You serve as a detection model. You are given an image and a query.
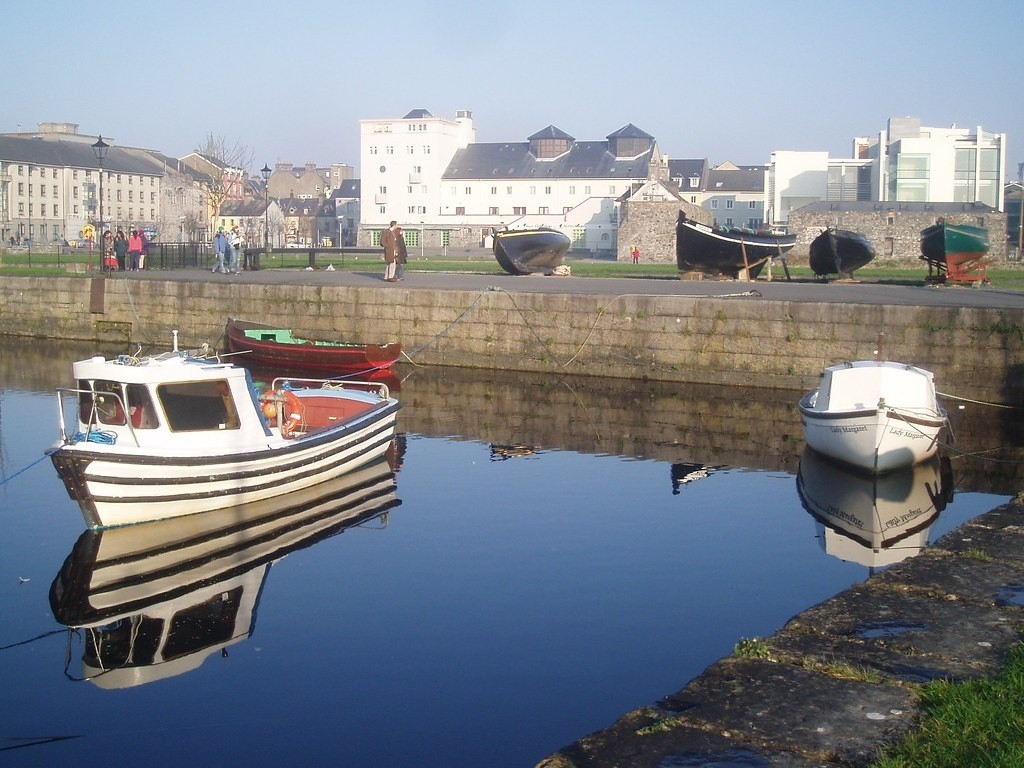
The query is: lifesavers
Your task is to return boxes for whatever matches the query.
[257,389,302,431]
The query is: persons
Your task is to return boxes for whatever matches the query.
[103,229,147,272]
[633,247,639,264]
[380,221,409,282]
[9,234,21,246]
[211,225,241,276]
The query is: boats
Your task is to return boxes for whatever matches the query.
[490,225,571,275]
[41,326,403,532]
[917,214,992,275]
[674,209,798,281]
[798,360,951,473]
[809,227,876,279]
[226,354,397,392]
[795,443,953,567]
[225,315,403,371]
[48,454,404,689]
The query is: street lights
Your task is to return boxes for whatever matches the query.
[90,134,109,273]
[419,220,425,255]
[260,163,272,247]
[337,215,344,255]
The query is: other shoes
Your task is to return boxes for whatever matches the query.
[384,278,404,282]
[236,271,240,273]
[226,268,229,272]
[212,268,215,272]
[221,270,226,274]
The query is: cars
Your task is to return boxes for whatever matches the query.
[75,239,97,248]
[283,240,311,248]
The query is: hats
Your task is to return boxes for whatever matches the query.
[218,226,224,230]
[139,230,144,234]
